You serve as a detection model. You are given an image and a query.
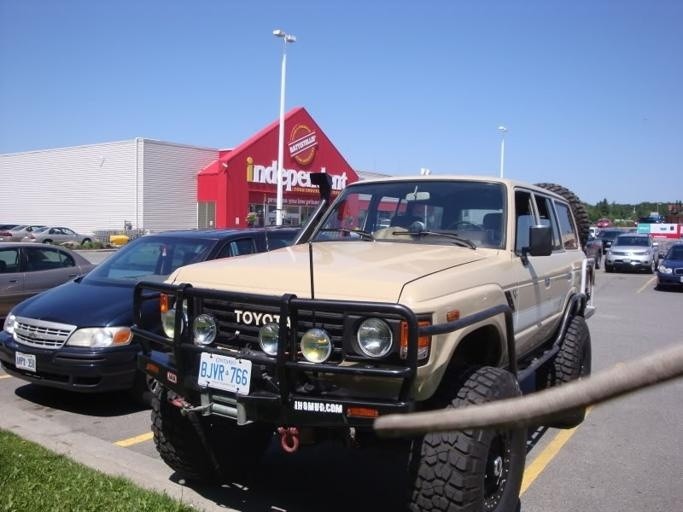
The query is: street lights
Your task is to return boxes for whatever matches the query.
[273,29,296,225]
[496,127,508,179]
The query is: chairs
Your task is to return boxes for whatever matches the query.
[483,213,501,243]
[391,216,423,229]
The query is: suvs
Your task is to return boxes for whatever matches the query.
[130,169,595,511]
[0,227,330,409]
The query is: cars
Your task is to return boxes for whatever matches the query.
[0,224,19,231]
[0,226,50,242]
[0,241,92,321]
[586,223,683,290]
[19,227,95,248]
